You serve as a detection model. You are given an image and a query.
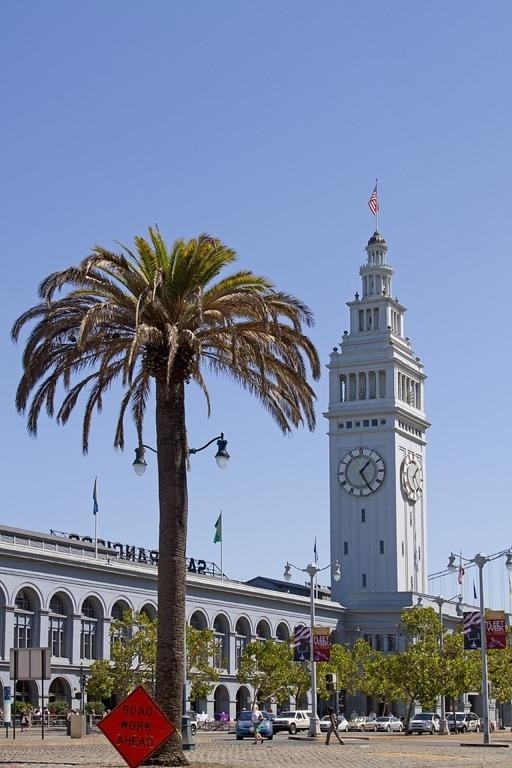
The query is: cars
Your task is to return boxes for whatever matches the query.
[347,716,378,732]
[320,714,349,733]
[479,719,494,733]
[398,716,412,722]
[234,711,275,740]
[268,713,277,723]
[445,712,457,719]
[374,717,403,733]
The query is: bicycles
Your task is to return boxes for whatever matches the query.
[210,713,231,731]
[196,717,212,730]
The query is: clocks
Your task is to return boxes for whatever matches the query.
[400,454,423,504]
[337,447,385,497]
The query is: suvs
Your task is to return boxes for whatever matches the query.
[187,711,197,736]
[272,710,321,735]
[445,712,480,734]
[408,713,441,734]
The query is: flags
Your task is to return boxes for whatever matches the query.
[474,585,477,598]
[214,514,222,544]
[368,184,378,215]
[314,540,318,560]
[93,480,99,515]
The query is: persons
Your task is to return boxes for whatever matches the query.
[325,707,345,745]
[251,704,264,745]
[19,706,50,732]
[350,709,394,719]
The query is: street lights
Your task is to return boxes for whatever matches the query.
[132,432,231,750]
[446,547,511,750]
[414,593,465,736]
[284,559,341,738]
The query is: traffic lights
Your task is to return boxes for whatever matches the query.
[81,674,86,687]
[324,673,336,692]
